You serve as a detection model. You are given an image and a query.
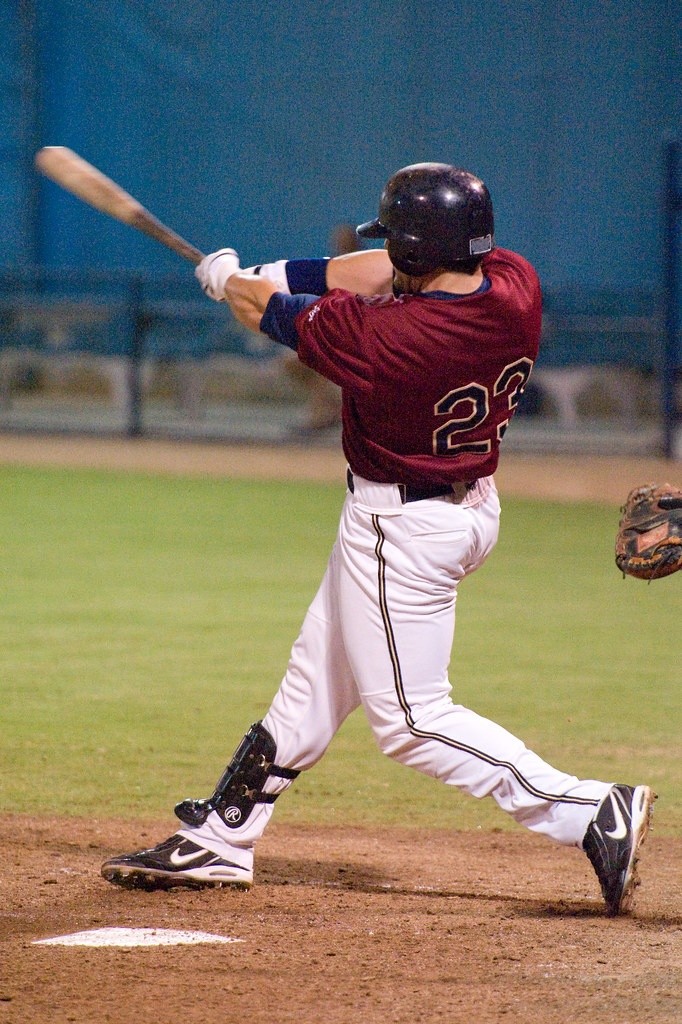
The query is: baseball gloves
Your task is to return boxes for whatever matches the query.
[613,483,681,582]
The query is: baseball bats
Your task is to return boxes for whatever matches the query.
[35,144,206,264]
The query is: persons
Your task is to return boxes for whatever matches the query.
[102,161,658,914]
[285,224,363,427]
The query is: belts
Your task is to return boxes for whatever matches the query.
[346,468,475,504]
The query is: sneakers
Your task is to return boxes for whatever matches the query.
[101,835,254,890]
[582,785,659,914]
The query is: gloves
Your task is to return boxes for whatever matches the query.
[194,248,291,302]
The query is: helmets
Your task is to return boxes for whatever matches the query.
[356,162,495,277]
